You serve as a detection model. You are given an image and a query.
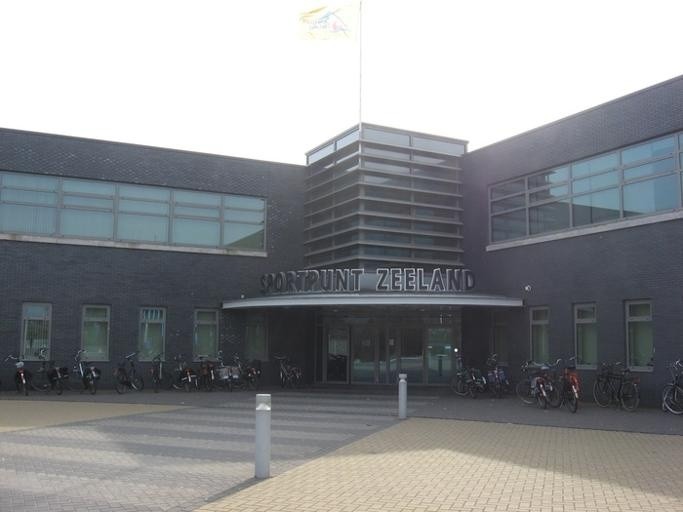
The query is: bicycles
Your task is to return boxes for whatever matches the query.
[5,352,28,395]
[74,349,99,396]
[446,346,683,417]
[172,350,262,394]
[30,347,69,395]
[113,350,146,395]
[276,357,302,390]
[150,351,167,393]
